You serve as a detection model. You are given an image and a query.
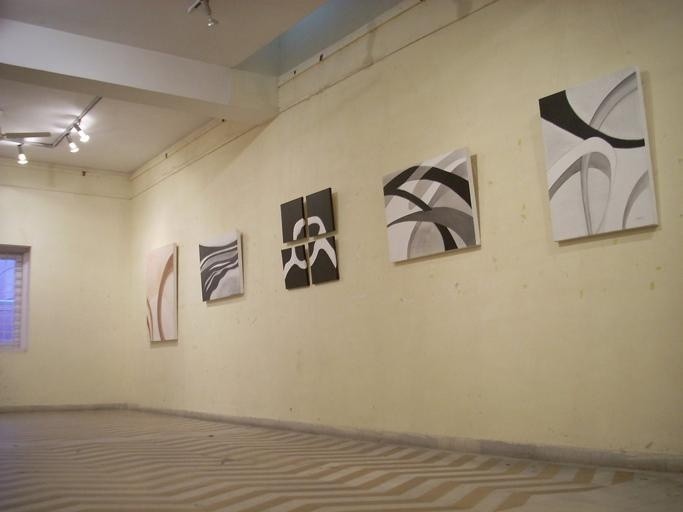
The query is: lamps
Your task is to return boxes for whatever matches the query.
[200,1,218,32]
